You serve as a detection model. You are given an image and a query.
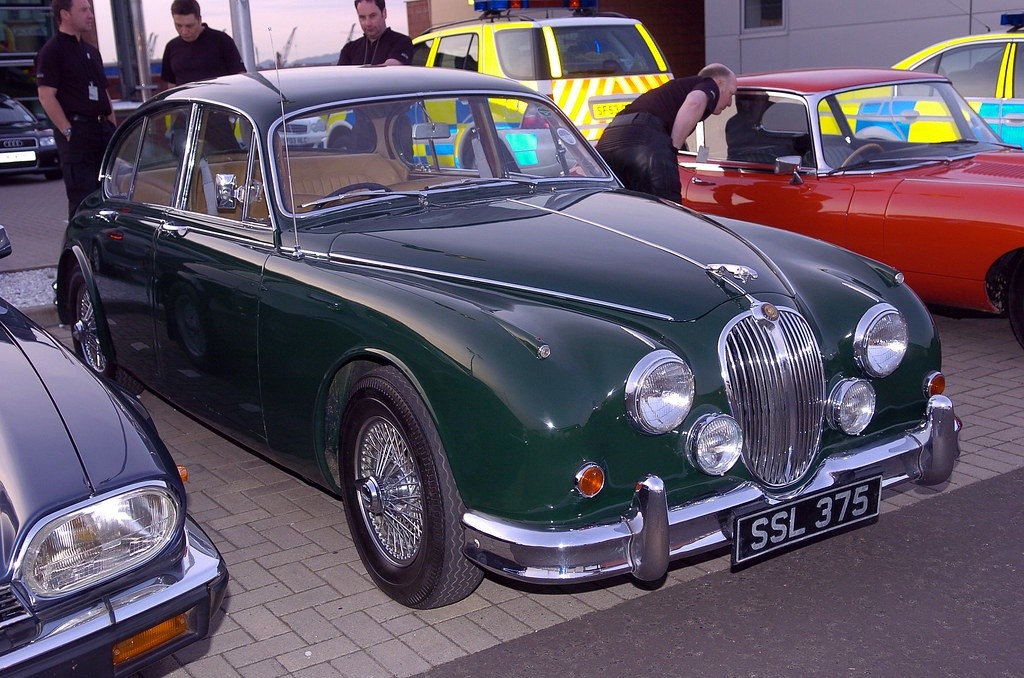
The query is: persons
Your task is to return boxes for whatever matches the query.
[596,62,737,207]
[725,85,806,164]
[160,0,247,159]
[33,0,117,221]
[338,0,415,165]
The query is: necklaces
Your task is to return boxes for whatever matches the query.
[362,37,381,65]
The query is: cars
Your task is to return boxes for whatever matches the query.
[278,114,330,150]
[55,63,960,611]
[0,91,65,182]
[676,69,1024,352]
[0,224,229,678]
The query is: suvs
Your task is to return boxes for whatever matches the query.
[323,0,679,178]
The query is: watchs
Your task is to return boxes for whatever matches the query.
[63,127,72,136]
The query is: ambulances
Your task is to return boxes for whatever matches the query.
[819,11,1024,148]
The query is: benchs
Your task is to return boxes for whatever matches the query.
[116,152,409,223]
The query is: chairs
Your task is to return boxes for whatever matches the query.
[823,146,865,168]
[748,145,781,165]
